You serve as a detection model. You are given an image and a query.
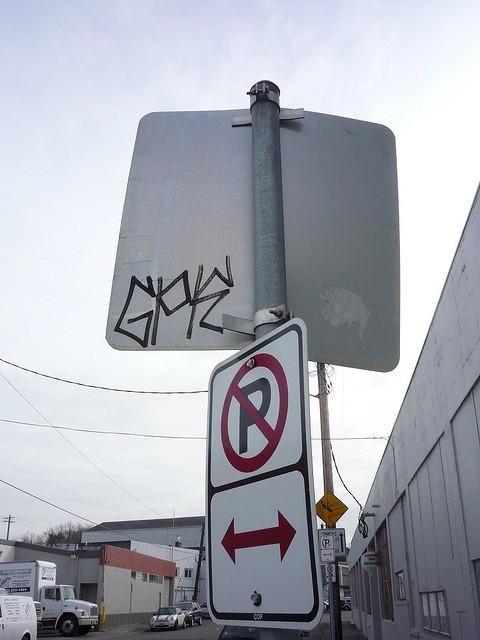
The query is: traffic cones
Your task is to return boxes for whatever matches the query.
[100,605,106,631]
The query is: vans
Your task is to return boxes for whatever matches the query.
[0,596,38,640]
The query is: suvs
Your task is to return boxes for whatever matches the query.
[340,597,351,611]
[174,600,203,627]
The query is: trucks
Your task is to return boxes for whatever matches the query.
[0,560,99,636]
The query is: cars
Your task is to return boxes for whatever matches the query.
[200,601,210,619]
[150,606,186,631]
